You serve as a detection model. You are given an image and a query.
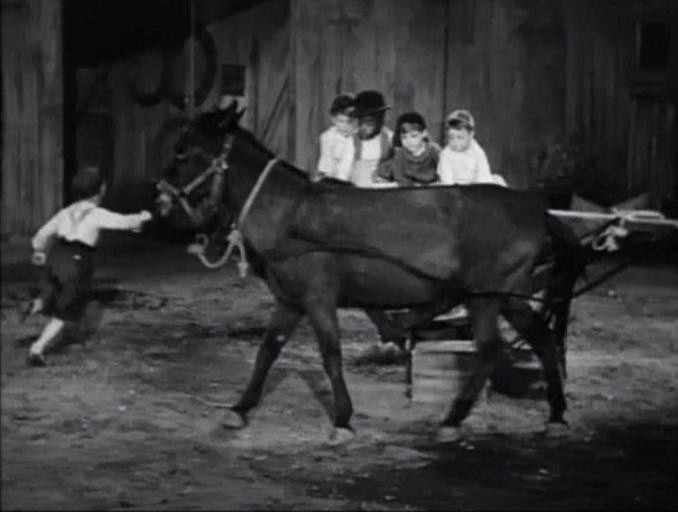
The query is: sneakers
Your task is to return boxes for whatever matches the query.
[19,299,34,321]
[27,350,45,364]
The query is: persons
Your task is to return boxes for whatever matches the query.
[437,111,500,186]
[381,112,444,187]
[17,167,155,366]
[337,89,398,189]
[311,92,358,182]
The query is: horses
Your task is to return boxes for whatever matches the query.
[150,99,583,450]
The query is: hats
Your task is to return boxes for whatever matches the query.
[348,90,389,118]
[446,109,474,128]
